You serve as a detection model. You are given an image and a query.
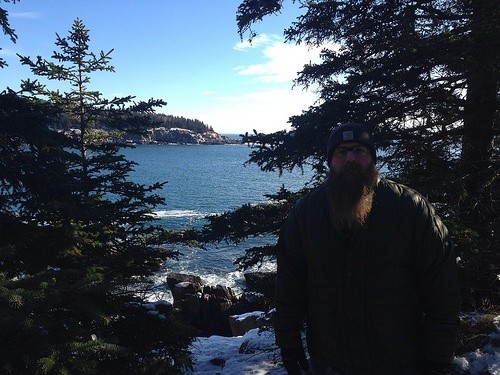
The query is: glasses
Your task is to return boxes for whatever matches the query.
[330,145,370,157]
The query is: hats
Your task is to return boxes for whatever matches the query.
[326,123,377,165]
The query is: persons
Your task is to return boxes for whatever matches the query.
[272,122,466,375]
